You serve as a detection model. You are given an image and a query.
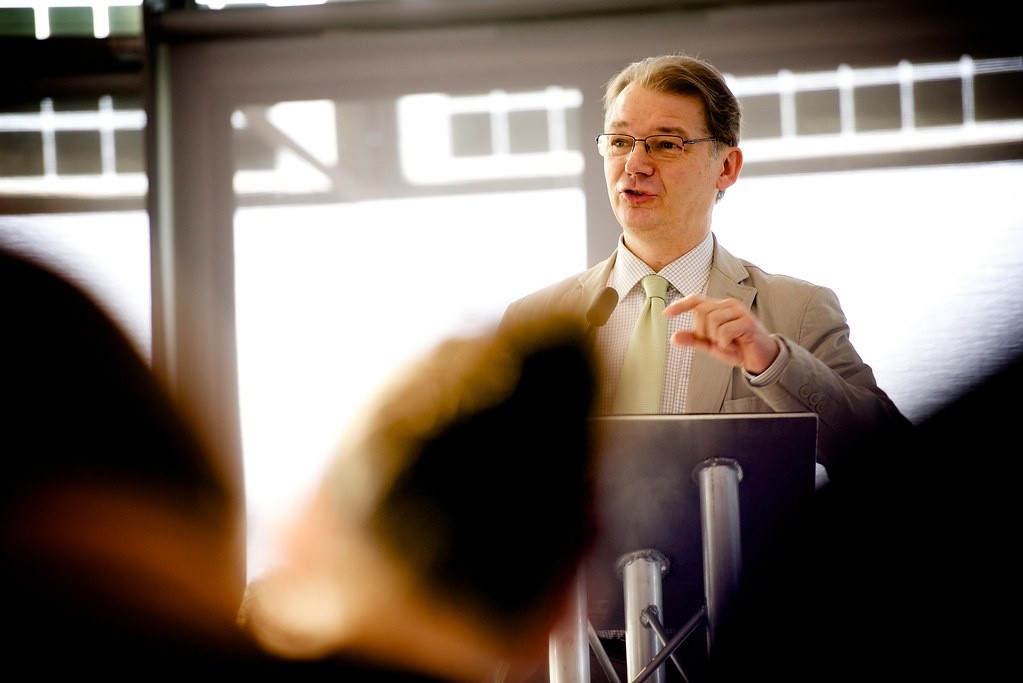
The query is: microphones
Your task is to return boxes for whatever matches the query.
[582,287,618,341]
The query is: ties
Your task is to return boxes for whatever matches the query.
[610,275,672,416]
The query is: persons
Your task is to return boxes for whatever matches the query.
[0,239,604,682]
[494,57,899,463]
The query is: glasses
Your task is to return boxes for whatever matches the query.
[595,133,734,161]
